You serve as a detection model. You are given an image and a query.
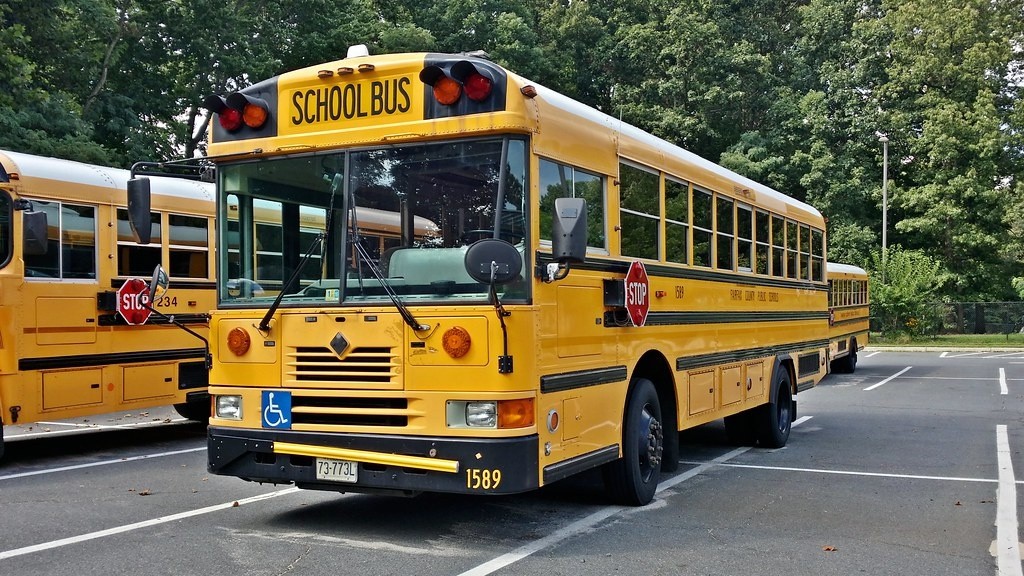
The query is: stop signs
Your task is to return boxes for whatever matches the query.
[624,259,649,328]
[116,278,155,326]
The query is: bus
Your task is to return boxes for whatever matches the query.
[0,150,441,465]
[127,43,830,507]
[827,262,871,374]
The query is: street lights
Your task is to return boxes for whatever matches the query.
[878,136,889,284]
